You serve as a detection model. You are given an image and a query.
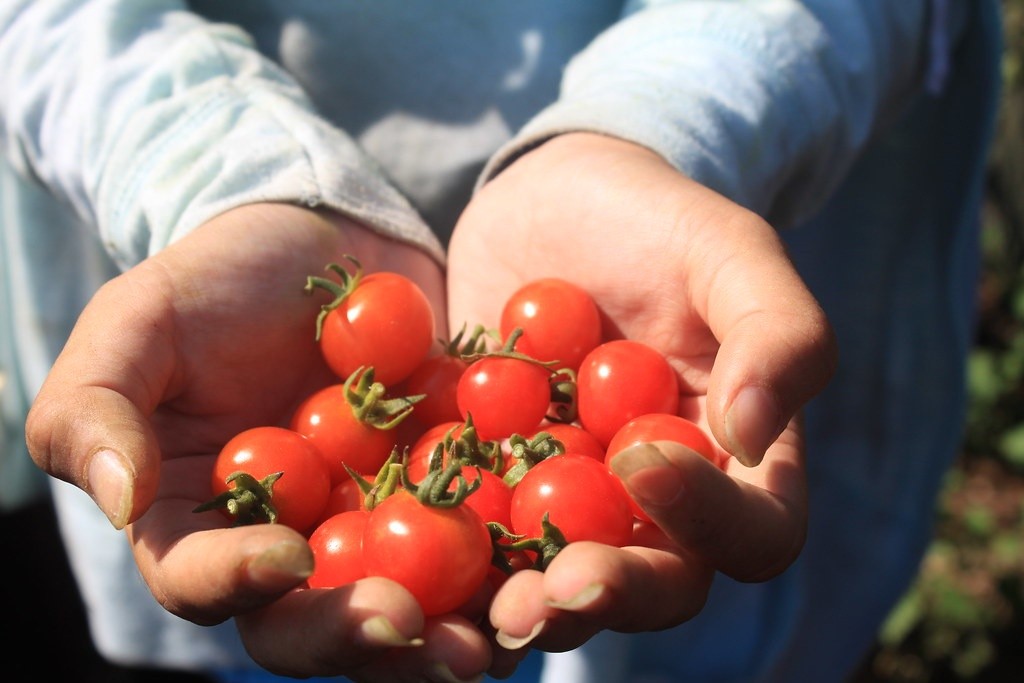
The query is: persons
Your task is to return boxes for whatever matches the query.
[1,1,1006,683]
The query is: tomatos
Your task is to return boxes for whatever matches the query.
[192,254,724,617]
